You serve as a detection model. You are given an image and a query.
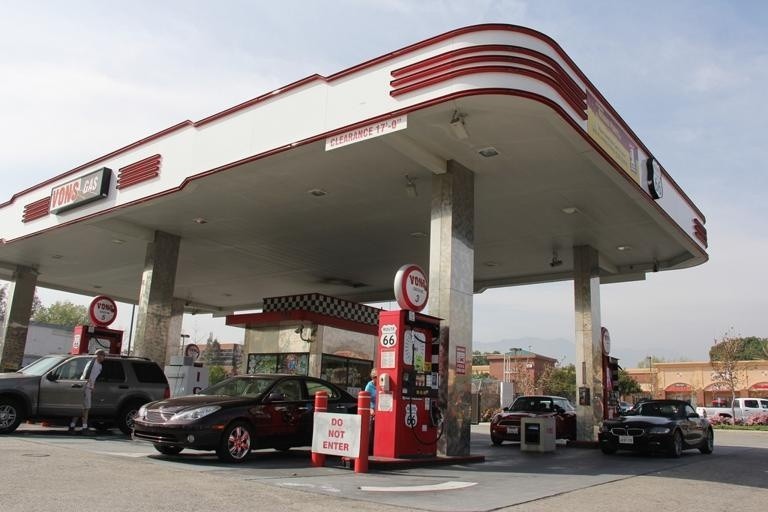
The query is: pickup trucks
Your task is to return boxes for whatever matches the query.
[695,398,768,427]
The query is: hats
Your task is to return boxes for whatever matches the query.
[95,349,107,355]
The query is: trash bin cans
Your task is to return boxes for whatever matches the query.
[520,416,557,452]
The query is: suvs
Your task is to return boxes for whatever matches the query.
[0,353,170,437]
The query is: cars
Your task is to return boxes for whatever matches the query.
[598,400,714,460]
[491,395,576,446]
[131,372,358,464]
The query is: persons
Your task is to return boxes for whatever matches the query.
[365,368,378,456]
[68,349,105,434]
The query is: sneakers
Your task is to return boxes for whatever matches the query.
[68,426,76,435]
[80,430,95,435]
